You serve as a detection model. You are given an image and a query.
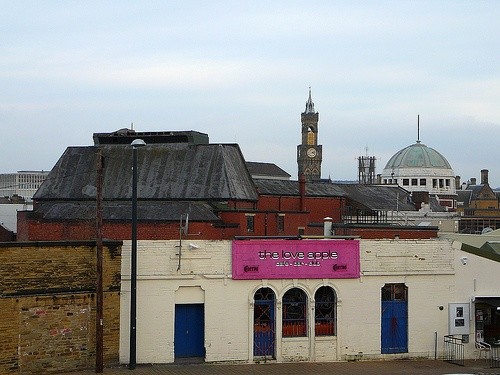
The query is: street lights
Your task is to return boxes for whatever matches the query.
[129,139,146,369]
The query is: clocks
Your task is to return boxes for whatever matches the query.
[306,147,317,158]
